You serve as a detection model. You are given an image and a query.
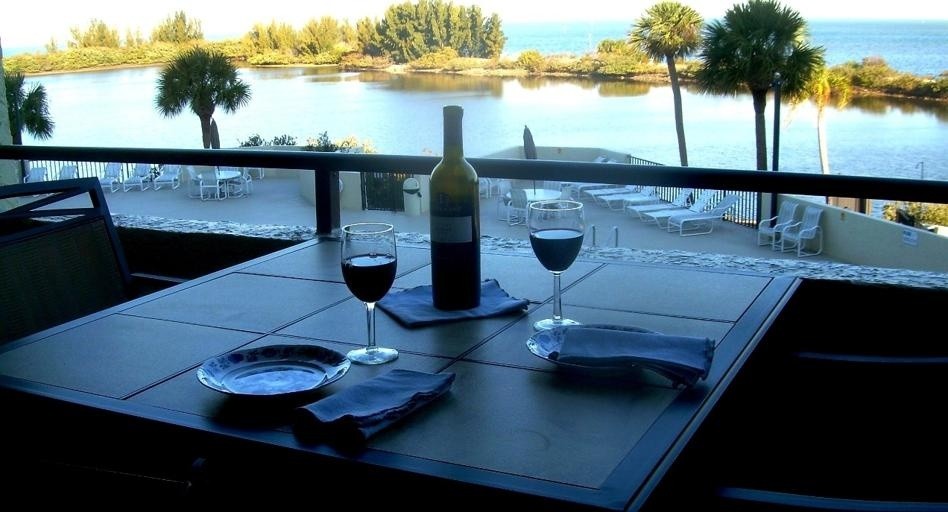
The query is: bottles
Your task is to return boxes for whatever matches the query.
[429,105,483,311]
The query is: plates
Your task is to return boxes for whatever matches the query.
[196,343,352,398]
[526,323,668,378]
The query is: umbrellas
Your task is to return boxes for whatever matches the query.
[210,119,221,172]
[522,125,538,196]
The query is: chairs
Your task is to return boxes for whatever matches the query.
[781,206,824,258]
[645,190,717,230]
[0,176,199,511]
[667,195,739,236]
[21,163,266,202]
[626,187,694,223]
[757,201,800,252]
[478,177,585,229]
[724,336,947,512]
[560,156,658,212]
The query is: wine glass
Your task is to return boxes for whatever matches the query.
[339,222,400,367]
[528,200,586,332]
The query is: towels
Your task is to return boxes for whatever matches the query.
[290,369,456,451]
[376,278,531,329]
[547,326,715,387]
